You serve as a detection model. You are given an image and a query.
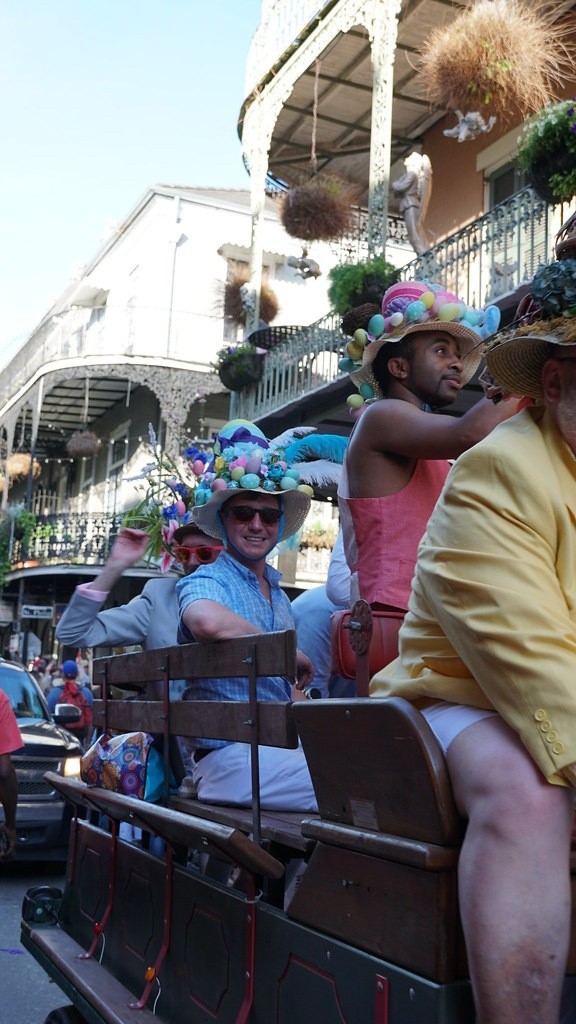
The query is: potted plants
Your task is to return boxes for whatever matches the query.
[326,247,402,336]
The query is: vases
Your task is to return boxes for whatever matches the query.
[219,352,263,392]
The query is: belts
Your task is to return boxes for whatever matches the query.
[193,749,214,764]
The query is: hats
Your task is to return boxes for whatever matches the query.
[60,659,78,673]
[193,447,314,541]
[158,479,212,545]
[337,279,500,417]
[485,258,576,399]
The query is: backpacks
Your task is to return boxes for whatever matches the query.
[55,681,93,728]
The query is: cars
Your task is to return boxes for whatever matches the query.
[21,596,576,1024]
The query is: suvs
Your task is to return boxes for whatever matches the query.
[0,657,86,862]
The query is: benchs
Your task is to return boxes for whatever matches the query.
[93,629,323,851]
[292,599,576,986]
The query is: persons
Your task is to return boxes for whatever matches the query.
[391,151,435,273]
[367,258,576,1024]
[55,511,227,773]
[339,282,529,701]
[0,689,28,858]
[48,660,94,747]
[168,421,351,814]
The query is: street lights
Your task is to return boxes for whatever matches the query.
[14,449,48,659]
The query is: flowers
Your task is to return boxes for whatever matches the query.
[510,98,576,209]
[209,341,263,385]
[121,413,212,574]
[530,257,576,318]
[194,442,285,505]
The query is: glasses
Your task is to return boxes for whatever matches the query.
[173,546,224,563]
[219,506,285,525]
